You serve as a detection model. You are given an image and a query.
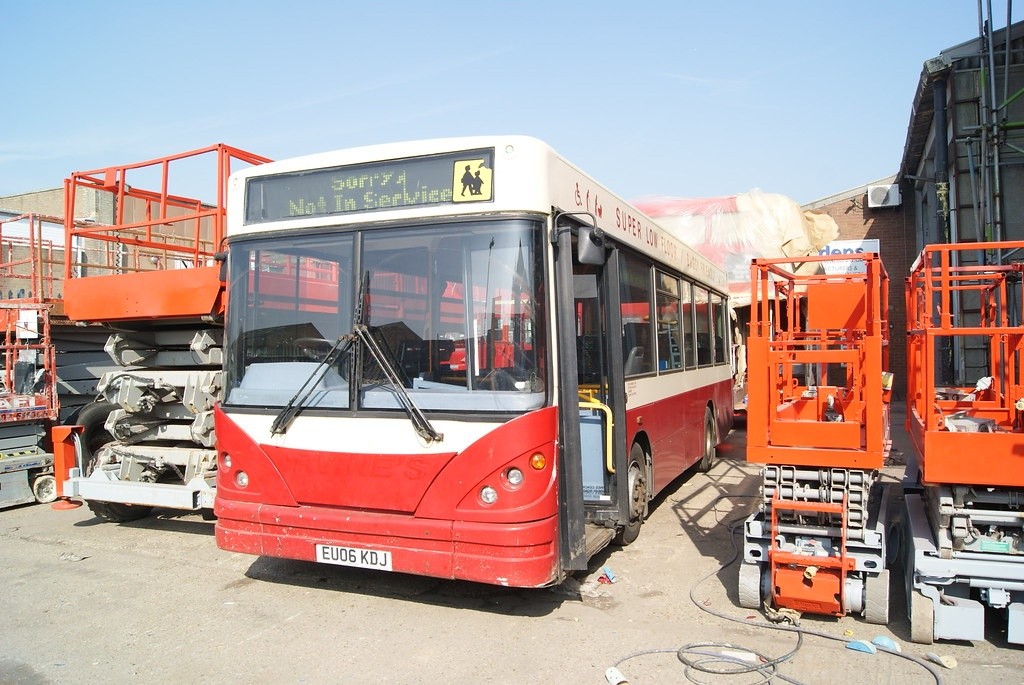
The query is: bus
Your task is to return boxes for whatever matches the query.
[215,134,736,588]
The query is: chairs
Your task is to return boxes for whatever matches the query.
[624,346,646,376]
